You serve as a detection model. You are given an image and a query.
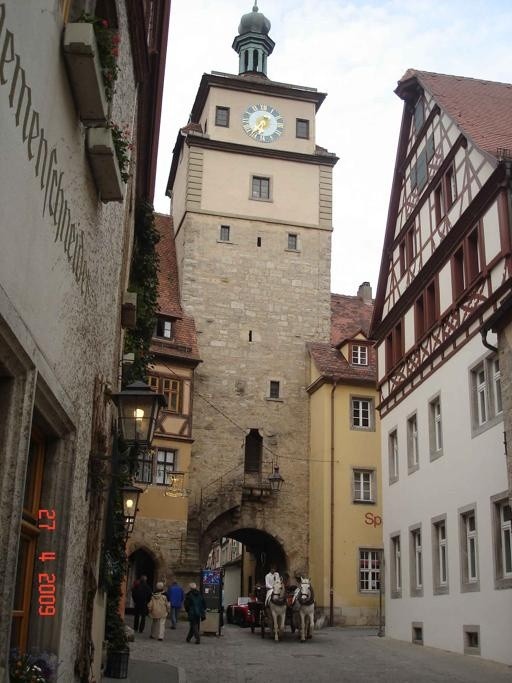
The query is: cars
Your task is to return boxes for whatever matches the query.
[227,596,258,629]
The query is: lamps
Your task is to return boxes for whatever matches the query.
[268,454,284,492]
[104,377,168,447]
[112,479,143,543]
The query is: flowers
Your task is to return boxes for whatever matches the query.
[74,8,121,104]
[10,645,63,682]
[111,120,135,183]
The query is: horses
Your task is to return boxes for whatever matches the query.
[266,576,288,643]
[292,576,315,643]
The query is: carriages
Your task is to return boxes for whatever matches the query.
[247,566,319,643]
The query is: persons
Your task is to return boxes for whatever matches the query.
[130,574,152,632]
[147,581,170,640]
[183,581,208,646]
[131,575,143,616]
[166,578,185,630]
[265,565,282,590]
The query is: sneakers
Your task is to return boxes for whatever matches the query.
[186,637,200,644]
[149,635,163,642]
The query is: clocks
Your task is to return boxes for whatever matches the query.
[240,102,283,143]
